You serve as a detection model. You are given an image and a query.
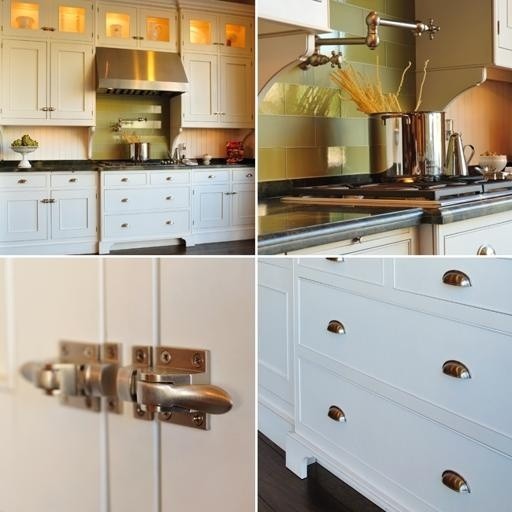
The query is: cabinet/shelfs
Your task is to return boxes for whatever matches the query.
[279,209,512,256]
[1,0,254,129]
[256,1,332,36]
[1,168,255,256]
[414,0,511,72]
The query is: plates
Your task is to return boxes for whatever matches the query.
[1,0,254,129]
[279,209,512,256]
[1,168,255,256]
[414,0,511,72]
[256,1,332,36]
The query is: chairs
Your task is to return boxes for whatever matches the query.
[257,259,511,512]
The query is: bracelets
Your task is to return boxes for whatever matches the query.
[481,155,507,173]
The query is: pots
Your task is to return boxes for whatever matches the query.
[128,142,149,162]
[368,110,447,181]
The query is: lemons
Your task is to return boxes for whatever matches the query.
[444,131,475,178]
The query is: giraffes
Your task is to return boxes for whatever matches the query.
[294,169,512,199]
[97,159,185,169]
